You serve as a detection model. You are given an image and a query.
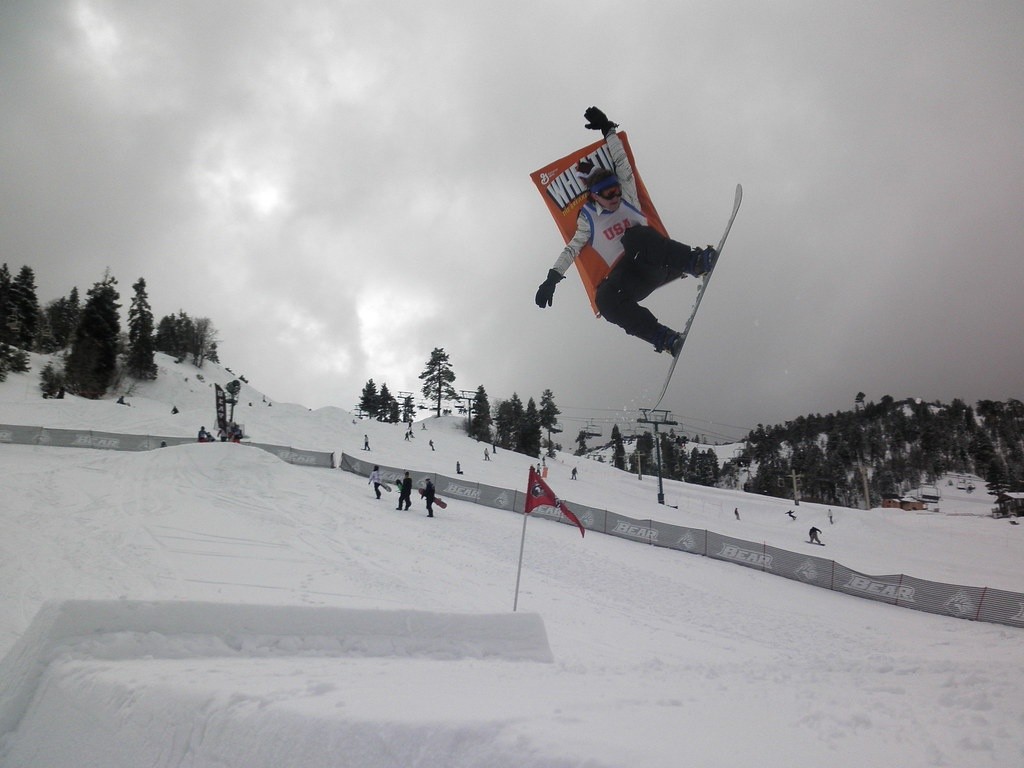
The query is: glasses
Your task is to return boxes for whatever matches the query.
[598,192,622,199]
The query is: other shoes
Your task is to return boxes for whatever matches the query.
[427,515,433,517]
[404,508,408,510]
[396,507,402,510]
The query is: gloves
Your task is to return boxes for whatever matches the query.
[535,269,566,308]
[584,106,615,137]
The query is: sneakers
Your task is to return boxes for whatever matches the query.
[688,248,716,277]
[661,329,685,356]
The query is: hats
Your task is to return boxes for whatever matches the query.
[578,161,620,192]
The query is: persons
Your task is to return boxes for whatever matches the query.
[809,527,822,544]
[429,440,435,451]
[455,461,463,475]
[196,418,243,443]
[534,105,717,357]
[396,472,412,511]
[571,467,579,480]
[368,466,382,499]
[404,408,467,441]
[735,508,741,520]
[171,406,179,415]
[536,463,542,476]
[493,441,496,454]
[421,478,436,517]
[364,435,370,451]
[116,396,133,406]
[484,448,490,460]
[828,509,834,524]
[785,510,796,520]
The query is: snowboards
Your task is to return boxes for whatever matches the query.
[395,479,411,507]
[646,184,743,416]
[805,541,825,546]
[419,488,447,510]
[381,482,392,492]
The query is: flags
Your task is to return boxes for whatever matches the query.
[523,469,584,538]
